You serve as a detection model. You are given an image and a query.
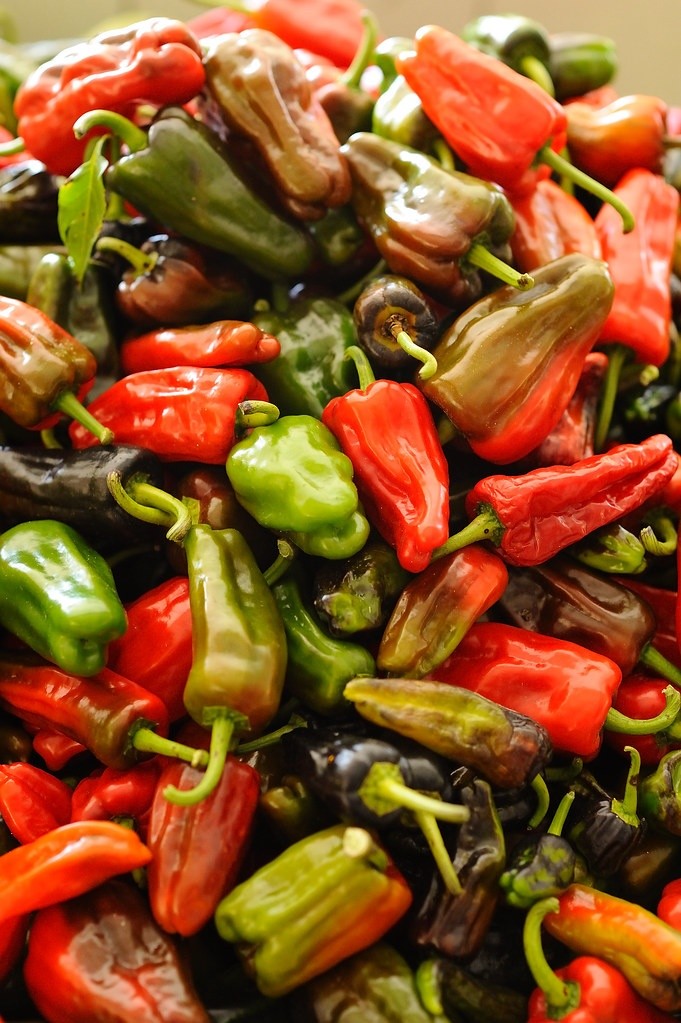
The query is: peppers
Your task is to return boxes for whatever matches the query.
[0,0,681,1023]
